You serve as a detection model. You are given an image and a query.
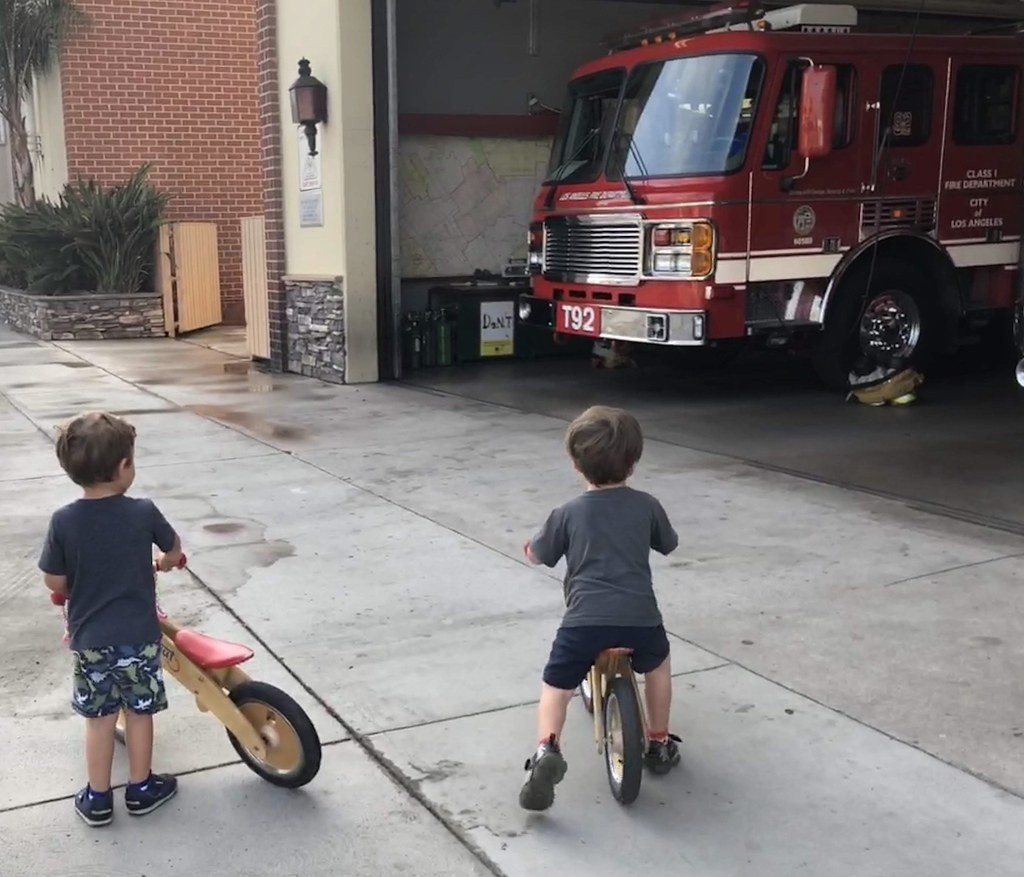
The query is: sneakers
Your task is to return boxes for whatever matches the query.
[644,734,683,777]
[125,769,178,816]
[520,733,569,812]
[75,783,114,828]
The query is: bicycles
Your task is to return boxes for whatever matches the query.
[523,542,647,806]
[48,552,322,788]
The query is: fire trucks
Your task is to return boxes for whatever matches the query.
[511,0,1024,386]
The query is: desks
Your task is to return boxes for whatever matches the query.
[445,286,525,355]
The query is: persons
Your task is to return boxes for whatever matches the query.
[37,413,181,825]
[519,406,682,812]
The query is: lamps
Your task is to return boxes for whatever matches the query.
[288,55,328,157]
